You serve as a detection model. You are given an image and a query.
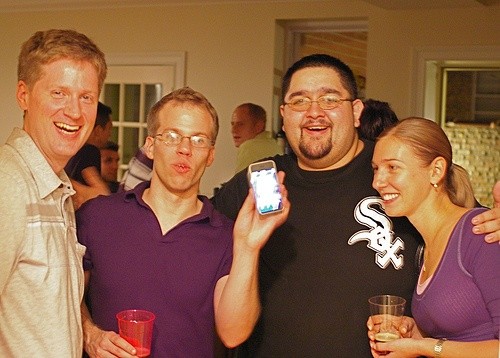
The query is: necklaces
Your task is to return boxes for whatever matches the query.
[423,246,431,272]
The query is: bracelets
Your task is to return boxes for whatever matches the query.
[434,339,445,358]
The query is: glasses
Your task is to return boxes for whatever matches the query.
[153,131,215,148]
[283,95,353,112]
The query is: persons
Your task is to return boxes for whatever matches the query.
[76,86,291,358]
[230,103,285,174]
[367,118,500,358]
[360,98,398,140]
[65,103,154,210]
[73,54,500,358]
[0,30,107,358]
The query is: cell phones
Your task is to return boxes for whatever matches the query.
[248,160,284,215]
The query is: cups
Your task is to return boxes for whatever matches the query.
[368,295,406,354]
[116,310,155,356]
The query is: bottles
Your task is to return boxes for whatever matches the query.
[275,117,285,155]
